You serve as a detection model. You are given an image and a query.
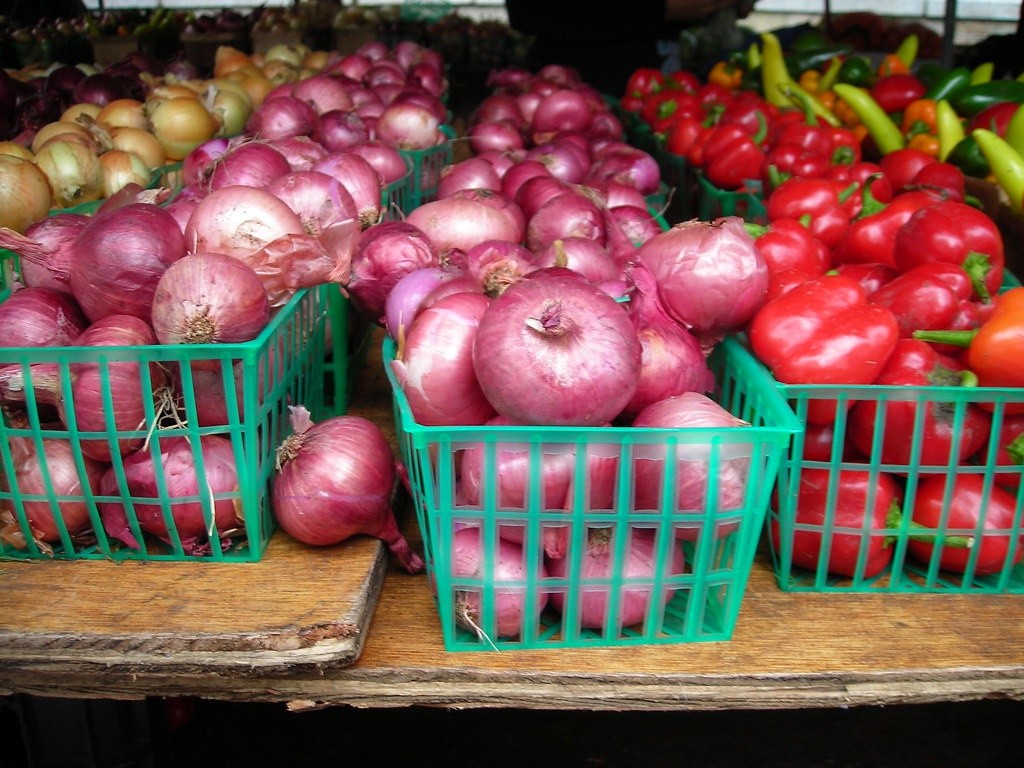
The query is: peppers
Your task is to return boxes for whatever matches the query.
[628,33,1024,580]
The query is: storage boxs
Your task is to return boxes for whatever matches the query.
[0,55,1023,653]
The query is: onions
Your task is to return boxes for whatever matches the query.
[0,42,764,628]
